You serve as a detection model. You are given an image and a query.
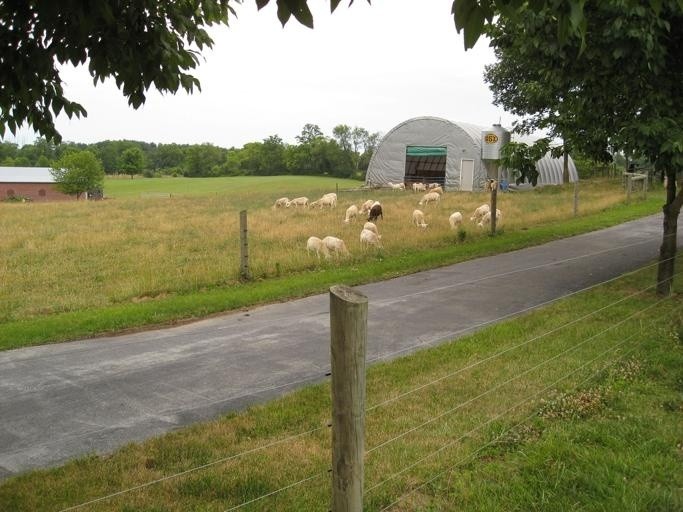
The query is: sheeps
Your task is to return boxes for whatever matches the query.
[344,200,384,225]
[412,209,428,230]
[478,209,500,230]
[270,193,337,212]
[360,229,384,254]
[322,235,353,261]
[387,182,444,206]
[364,221,383,242]
[470,204,490,224]
[307,236,333,262]
[449,212,462,230]
